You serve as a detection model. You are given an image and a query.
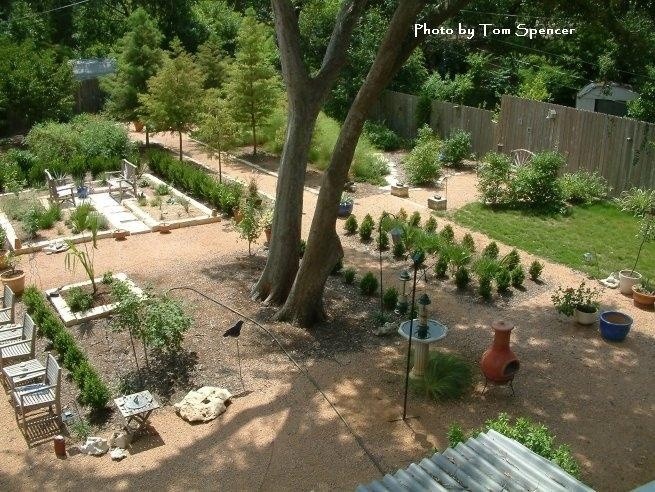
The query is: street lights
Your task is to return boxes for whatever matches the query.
[378,210,403,312]
[385,269,432,422]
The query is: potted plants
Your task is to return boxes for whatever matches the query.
[549,277,599,323]
[607,182,654,294]
[339,191,357,219]
[629,276,655,309]
[262,199,274,244]
[0,249,25,297]
[230,192,247,221]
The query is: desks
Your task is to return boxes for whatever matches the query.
[0,358,48,389]
[114,389,157,441]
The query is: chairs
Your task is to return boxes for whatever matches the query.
[0,310,39,378]
[11,349,66,445]
[0,285,18,329]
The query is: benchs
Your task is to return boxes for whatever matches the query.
[42,168,77,210]
[102,158,139,205]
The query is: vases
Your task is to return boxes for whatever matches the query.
[158,221,170,233]
[113,229,126,239]
[600,310,633,342]
[132,119,144,132]
